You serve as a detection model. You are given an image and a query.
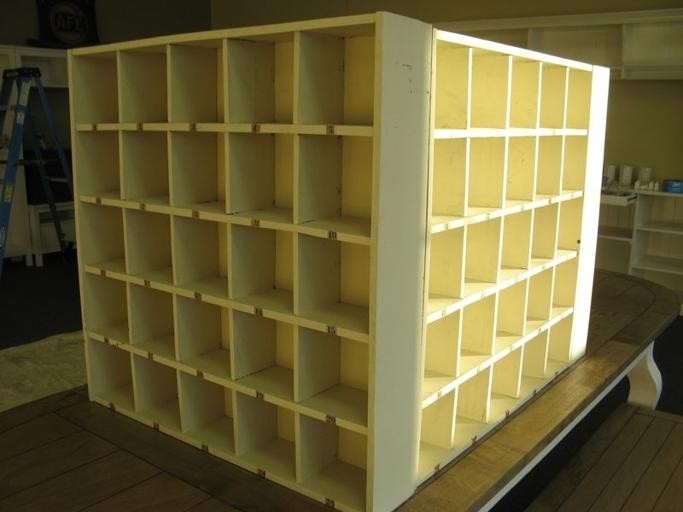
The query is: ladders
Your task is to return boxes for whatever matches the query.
[0,66,74,280]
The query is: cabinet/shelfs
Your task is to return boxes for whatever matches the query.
[19,49,77,265]
[417,28,609,489]
[597,191,633,243]
[67,8,433,510]
[629,189,682,317]
[2,47,34,270]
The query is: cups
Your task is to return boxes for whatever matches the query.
[619,165,635,186]
[637,165,652,183]
[605,164,617,185]
